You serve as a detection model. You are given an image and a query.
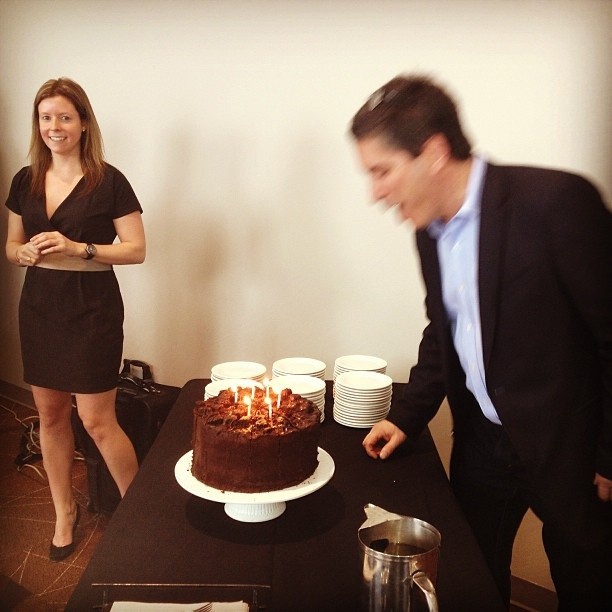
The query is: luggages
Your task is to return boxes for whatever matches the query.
[86,351,197,516]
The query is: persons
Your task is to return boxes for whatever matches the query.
[352,76,612,612]
[5,78,146,561]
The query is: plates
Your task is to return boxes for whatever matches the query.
[271,358,327,424]
[331,352,389,429]
[207,359,266,399]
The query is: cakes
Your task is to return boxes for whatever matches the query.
[173,378,336,523]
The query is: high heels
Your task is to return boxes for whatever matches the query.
[50,503,81,561]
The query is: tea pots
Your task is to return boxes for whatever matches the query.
[358,502,459,607]
